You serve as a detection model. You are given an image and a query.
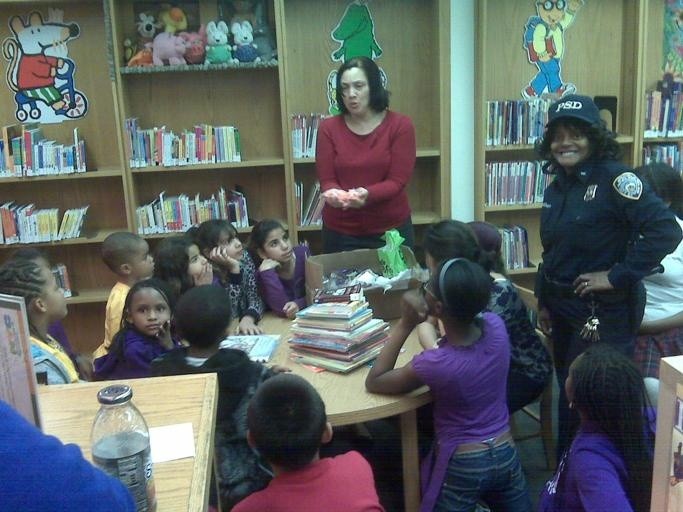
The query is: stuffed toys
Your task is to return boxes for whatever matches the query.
[122,7,278,67]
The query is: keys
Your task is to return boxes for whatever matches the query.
[579,315,601,342]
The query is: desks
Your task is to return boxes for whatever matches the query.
[185,312,444,510]
[34,371,222,512]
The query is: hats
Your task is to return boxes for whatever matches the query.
[544,95,600,130]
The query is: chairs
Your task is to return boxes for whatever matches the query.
[509,283,555,473]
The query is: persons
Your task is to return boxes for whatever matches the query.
[0,219,389,512]
[633,163,683,392]
[469,222,502,255]
[316,57,417,253]
[536,95,682,469]
[417,219,553,459]
[362,257,535,511]
[536,343,658,512]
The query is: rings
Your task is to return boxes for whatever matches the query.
[585,281,589,286]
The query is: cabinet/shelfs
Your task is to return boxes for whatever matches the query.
[648,355,683,512]
[280,0,451,246]
[108,0,294,252]
[474,0,643,267]
[636,0,683,168]
[0,2,133,357]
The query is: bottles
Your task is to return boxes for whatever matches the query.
[90,384,158,512]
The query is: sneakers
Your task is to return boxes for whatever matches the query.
[349,422,374,450]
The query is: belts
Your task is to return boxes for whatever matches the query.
[542,272,574,299]
[455,431,511,455]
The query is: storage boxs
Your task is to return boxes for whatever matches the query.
[303,245,422,321]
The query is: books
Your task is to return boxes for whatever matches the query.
[486,90,683,269]
[292,115,322,226]
[126,116,240,168]
[0,123,86,177]
[0,201,90,244]
[137,186,248,234]
